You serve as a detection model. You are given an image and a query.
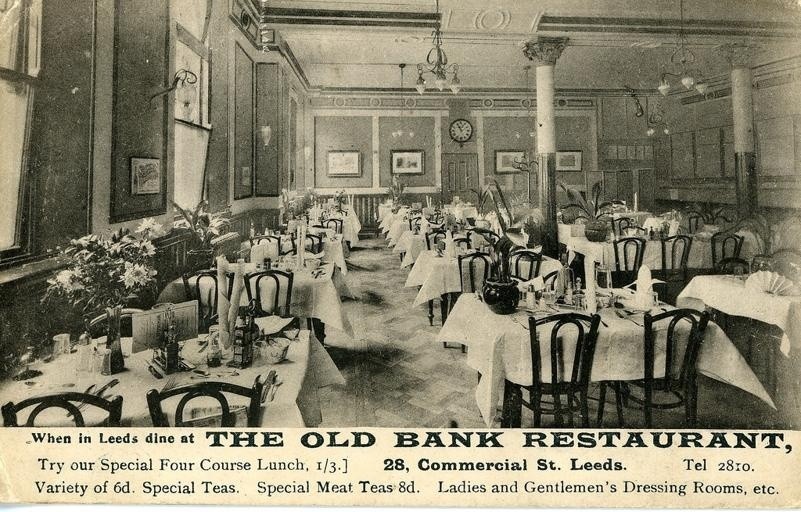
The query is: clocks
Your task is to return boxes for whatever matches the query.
[449,118,474,143]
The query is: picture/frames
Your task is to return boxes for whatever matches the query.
[390,148,425,175]
[494,149,527,175]
[129,155,163,195]
[555,149,583,172]
[326,149,362,177]
[241,166,252,187]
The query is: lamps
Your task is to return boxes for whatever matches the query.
[514,66,536,139]
[646,93,669,137]
[391,63,416,138]
[149,68,198,122]
[656,0,709,96]
[244,118,272,147]
[415,0,462,96]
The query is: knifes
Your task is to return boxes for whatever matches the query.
[66,378,119,417]
[260,370,276,403]
[198,340,208,353]
[44,349,79,362]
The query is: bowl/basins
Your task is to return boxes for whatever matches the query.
[258,338,290,365]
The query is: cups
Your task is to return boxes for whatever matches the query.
[460,241,468,255]
[53,333,73,386]
[732,266,745,288]
[237,255,321,276]
[206,344,222,366]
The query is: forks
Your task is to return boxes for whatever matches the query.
[510,314,530,330]
[161,377,176,395]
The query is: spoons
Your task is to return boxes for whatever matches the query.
[615,308,640,326]
[192,369,240,376]
[84,384,96,395]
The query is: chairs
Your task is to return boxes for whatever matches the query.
[383,201,800,428]
[0,210,349,427]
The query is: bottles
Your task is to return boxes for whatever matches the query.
[161,326,180,372]
[526,263,617,315]
[264,227,297,242]
[77,330,113,375]
[233,305,260,367]
[637,264,654,308]
[476,240,490,253]
[636,224,665,241]
[446,230,454,258]
